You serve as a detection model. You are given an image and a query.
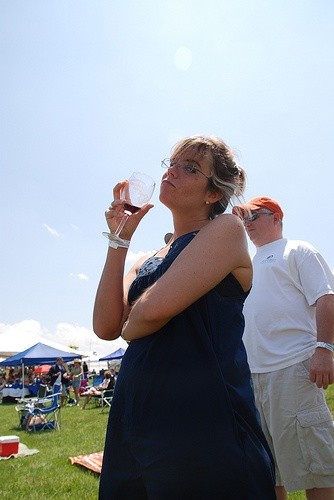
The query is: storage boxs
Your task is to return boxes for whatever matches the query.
[0,436,20,457]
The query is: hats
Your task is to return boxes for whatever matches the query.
[73,359,80,362]
[232,197,284,220]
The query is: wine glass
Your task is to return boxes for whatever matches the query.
[102,172,156,249]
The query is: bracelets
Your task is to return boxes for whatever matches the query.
[317,342,334,352]
[108,238,131,250]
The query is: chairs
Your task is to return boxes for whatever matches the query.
[67,376,115,413]
[16,384,62,435]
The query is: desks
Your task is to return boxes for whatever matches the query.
[3,387,30,404]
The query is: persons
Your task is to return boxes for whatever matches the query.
[232,196,334,500]
[93,134,278,500]
[0,358,117,407]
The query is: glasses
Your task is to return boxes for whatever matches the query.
[242,212,275,224]
[161,158,214,185]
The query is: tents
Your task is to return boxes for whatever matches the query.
[99,348,127,369]
[0,342,83,399]
